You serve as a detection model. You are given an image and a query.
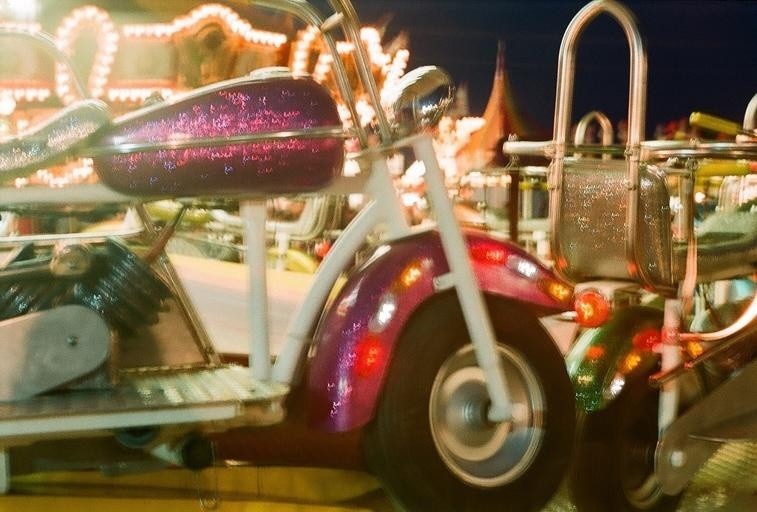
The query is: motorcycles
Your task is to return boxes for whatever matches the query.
[0,0,577,510]
[545,0,756,512]
